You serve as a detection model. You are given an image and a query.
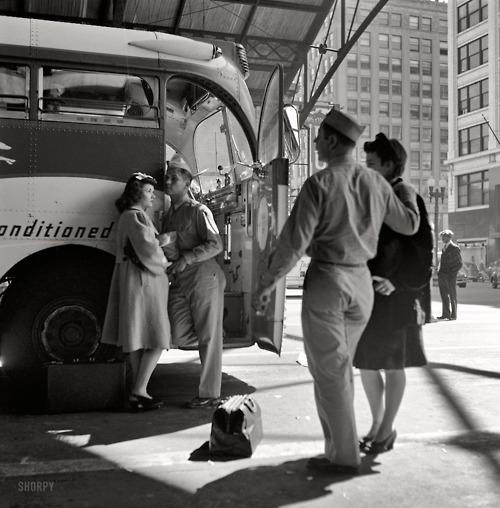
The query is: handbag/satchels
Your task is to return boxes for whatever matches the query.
[210,394,263,457]
[128,231,179,271]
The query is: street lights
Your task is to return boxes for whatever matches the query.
[428,177,447,269]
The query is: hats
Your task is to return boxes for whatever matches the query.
[441,229,454,237]
[323,106,366,143]
[167,154,193,178]
[377,132,407,166]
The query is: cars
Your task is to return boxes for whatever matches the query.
[434,270,467,287]
[490,259,500,287]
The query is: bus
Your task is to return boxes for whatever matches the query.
[0,12,300,411]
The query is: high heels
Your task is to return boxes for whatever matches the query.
[128,394,165,412]
[360,429,396,453]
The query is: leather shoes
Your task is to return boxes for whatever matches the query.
[437,316,456,320]
[186,397,221,407]
[306,458,358,473]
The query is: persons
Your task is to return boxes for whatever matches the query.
[411,282,437,323]
[161,157,226,409]
[252,107,421,474]
[100,172,173,412]
[438,229,462,321]
[353,133,433,453]
